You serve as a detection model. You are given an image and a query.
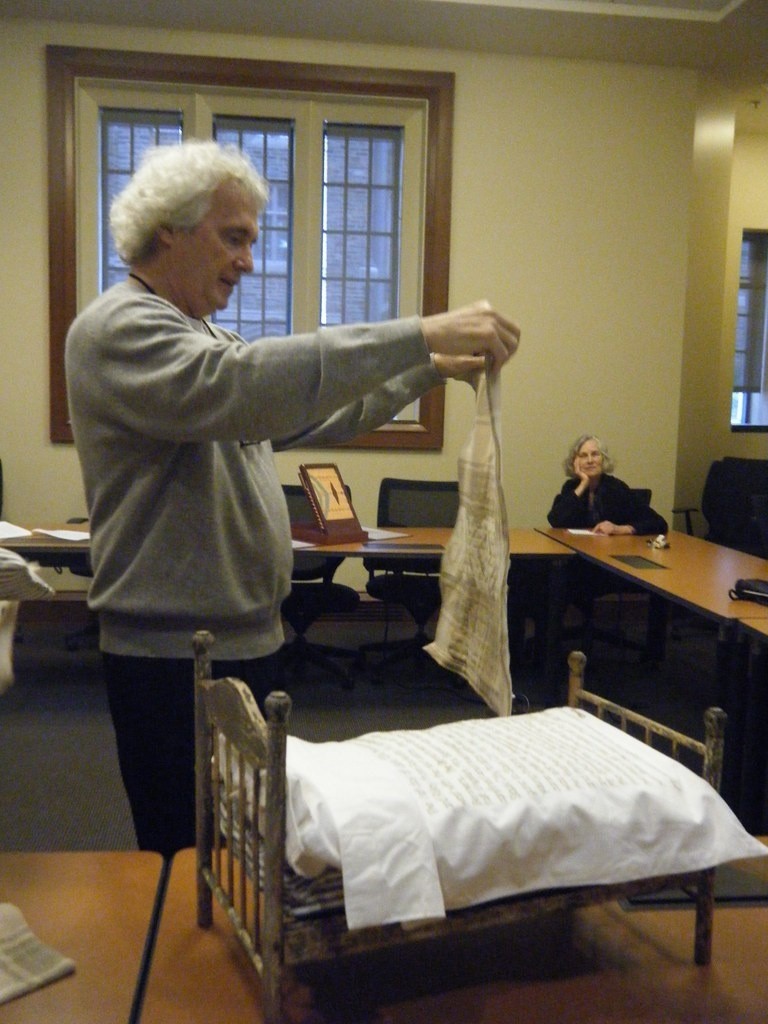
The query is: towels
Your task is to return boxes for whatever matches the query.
[420,352,515,718]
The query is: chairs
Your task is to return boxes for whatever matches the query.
[358,475,461,682]
[275,484,368,692]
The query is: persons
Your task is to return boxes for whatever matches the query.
[547,435,668,535]
[64,140,521,853]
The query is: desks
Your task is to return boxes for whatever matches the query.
[534,524,768,839]
[0,516,578,707]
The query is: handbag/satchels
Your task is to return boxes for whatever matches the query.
[729,579,768,606]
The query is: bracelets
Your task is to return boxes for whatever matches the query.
[627,525,634,534]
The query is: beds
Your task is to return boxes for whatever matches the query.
[198,626,728,1021]
